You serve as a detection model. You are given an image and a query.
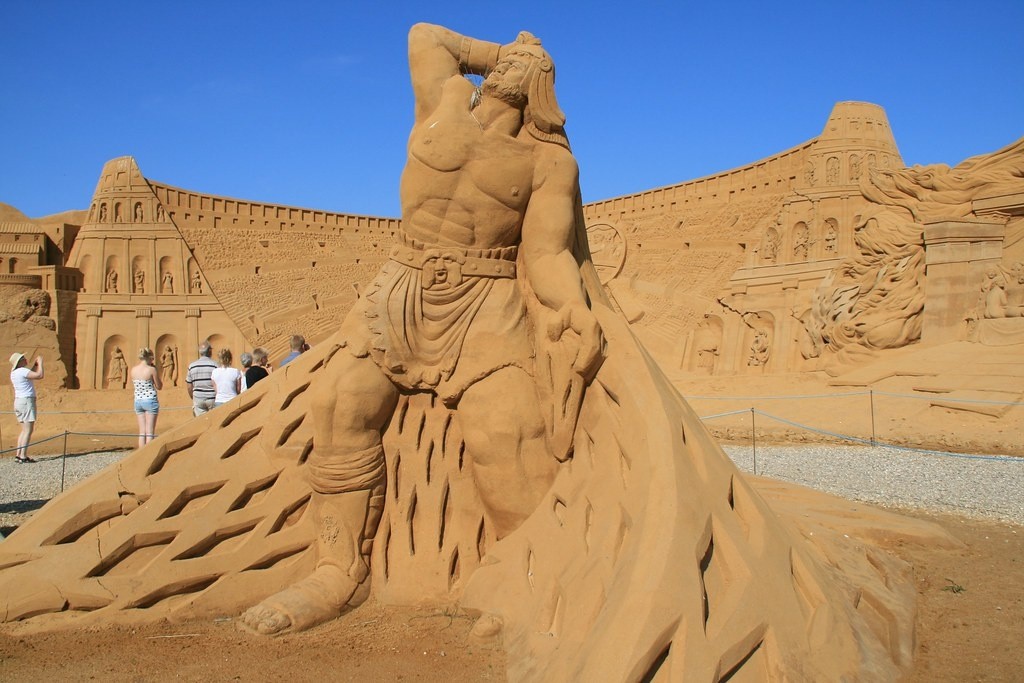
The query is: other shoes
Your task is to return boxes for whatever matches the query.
[14,456,20,463]
[18,457,36,464]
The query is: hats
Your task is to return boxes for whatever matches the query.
[9,352,27,371]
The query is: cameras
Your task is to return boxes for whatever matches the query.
[304,344,310,350]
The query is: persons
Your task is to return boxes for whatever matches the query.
[978,263,1024,318]
[108,269,117,288]
[134,269,144,288]
[794,234,807,256]
[130,347,163,446]
[162,271,173,288]
[238,22,604,635]
[100,205,165,222]
[763,237,775,258]
[184,334,311,417]
[192,271,201,288]
[825,227,836,253]
[160,345,177,380]
[107,346,126,381]
[9,351,44,464]
